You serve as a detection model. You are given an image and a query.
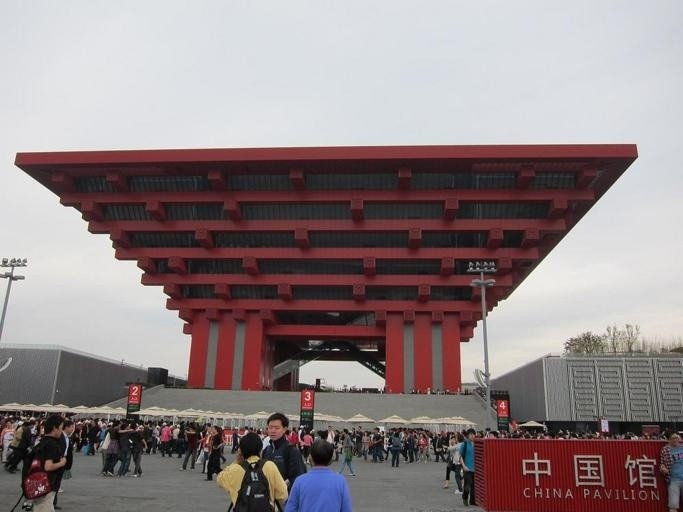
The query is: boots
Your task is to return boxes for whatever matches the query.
[444,480,449,488]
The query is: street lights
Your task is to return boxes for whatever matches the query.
[0,258,27,337]
[469,261,498,428]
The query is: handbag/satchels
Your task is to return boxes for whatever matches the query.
[22,471,53,499]
[342,448,353,462]
[447,458,455,471]
[435,448,443,452]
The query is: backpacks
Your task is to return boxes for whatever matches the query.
[235,459,275,512]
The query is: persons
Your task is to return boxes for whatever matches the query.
[0,414,683,512]
[344,387,472,395]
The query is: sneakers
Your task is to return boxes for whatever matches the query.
[179,467,195,471]
[3,462,20,473]
[455,490,463,494]
[463,499,478,506]
[100,469,143,478]
